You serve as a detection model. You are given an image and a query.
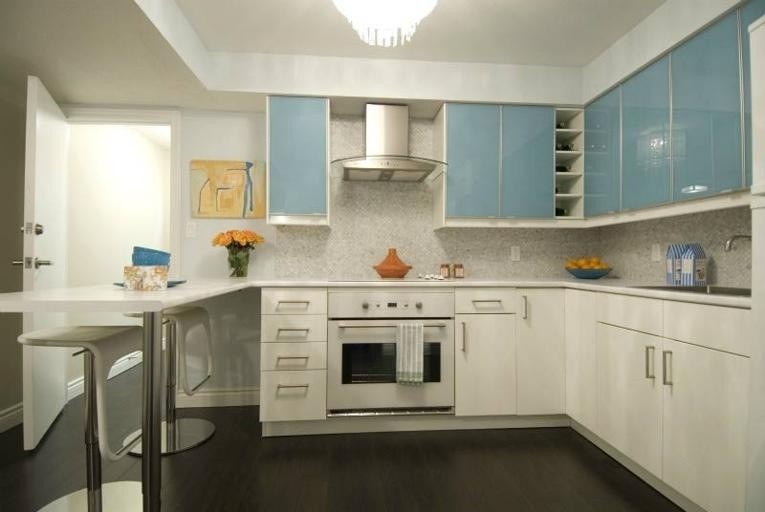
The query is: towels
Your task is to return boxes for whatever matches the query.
[395,323,426,386]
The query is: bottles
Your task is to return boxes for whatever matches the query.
[456,263,463,279]
[442,263,451,278]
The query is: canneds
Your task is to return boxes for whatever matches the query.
[440,263,450,278]
[454,263,465,278]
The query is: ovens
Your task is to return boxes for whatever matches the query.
[328,293,455,420]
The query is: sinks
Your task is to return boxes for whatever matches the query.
[626,285,751,296]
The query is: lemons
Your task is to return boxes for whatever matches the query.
[564,256,610,270]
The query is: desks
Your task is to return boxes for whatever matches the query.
[0,278,250,510]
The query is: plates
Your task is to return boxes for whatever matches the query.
[568,268,613,279]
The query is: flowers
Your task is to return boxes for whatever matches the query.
[212,229,266,279]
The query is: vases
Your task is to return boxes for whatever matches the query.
[225,243,252,283]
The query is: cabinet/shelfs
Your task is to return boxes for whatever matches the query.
[454,287,519,431]
[584,4,751,229]
[519,290,568,429]
[432,104,584,229]
[596,292,751,512]
[568,289,599,445]
[265,96,333,231]
[258,288,327,438]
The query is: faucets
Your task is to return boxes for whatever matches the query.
[725,235,752,251]
